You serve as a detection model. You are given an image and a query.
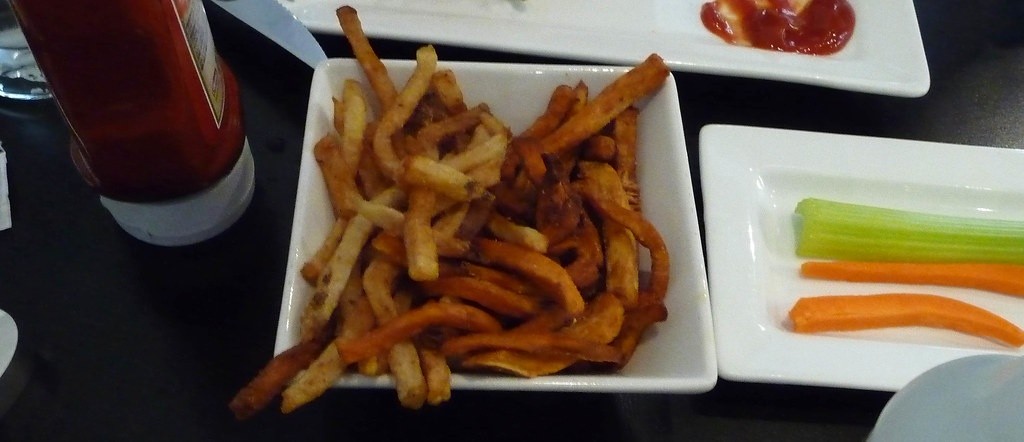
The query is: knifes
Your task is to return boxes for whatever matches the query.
[212,0,328,69]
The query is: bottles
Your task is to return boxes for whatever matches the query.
[7,0,256,247]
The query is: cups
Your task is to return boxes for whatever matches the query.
[0,0,52,100]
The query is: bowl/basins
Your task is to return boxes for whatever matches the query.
[864,354,1024,442]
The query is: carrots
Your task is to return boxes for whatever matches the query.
[789,292,1024,348]
[800,261,1024,298]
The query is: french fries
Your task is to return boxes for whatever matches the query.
[227,4,673,420]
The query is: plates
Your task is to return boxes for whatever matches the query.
[699,124,1024,392]
[277,0,931,98]
[276,58,717,394]
